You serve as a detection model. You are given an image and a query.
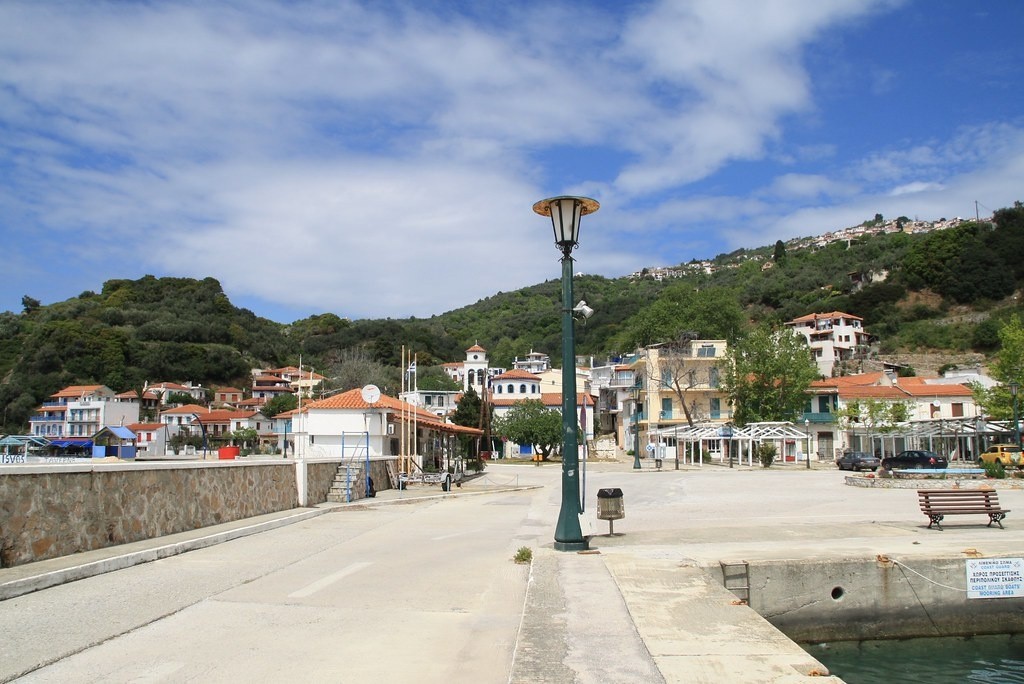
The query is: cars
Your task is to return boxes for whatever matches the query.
[880,450,948,472]
[837,450,881,472]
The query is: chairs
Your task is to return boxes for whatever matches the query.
[916,486,1011,531]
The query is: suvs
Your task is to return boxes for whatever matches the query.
[978,443,1024,470]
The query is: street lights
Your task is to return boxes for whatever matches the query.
[532,193,599,549]
[805,418,812,468]
[631,384,642,469]
[1011,381,1023,443]
